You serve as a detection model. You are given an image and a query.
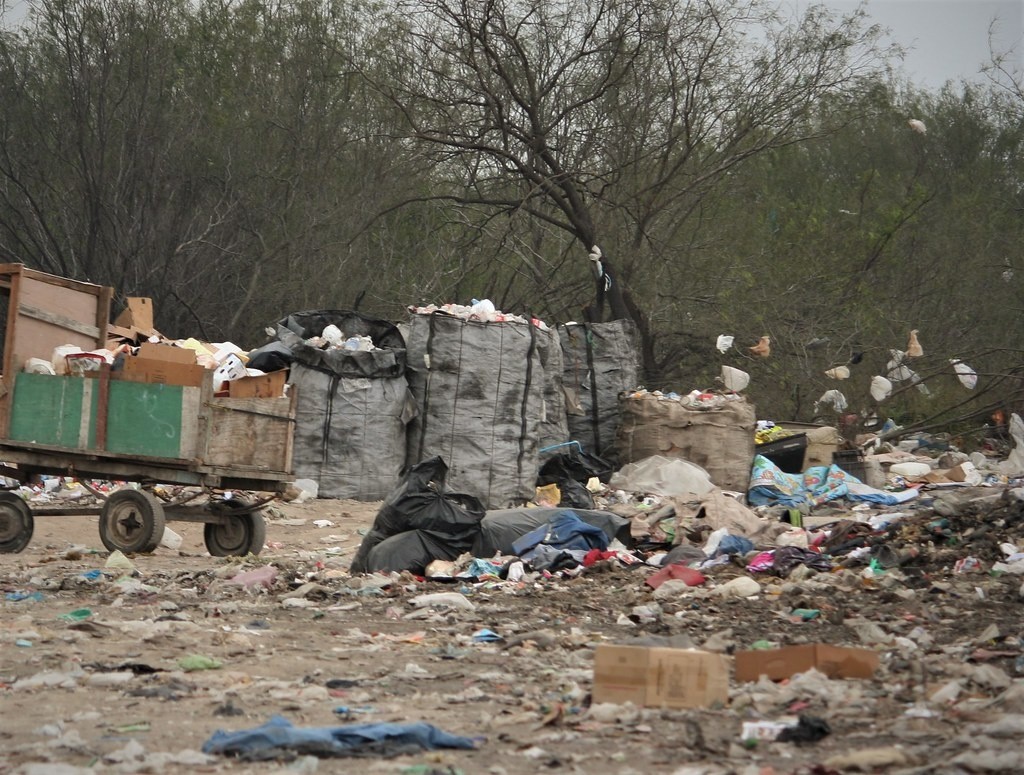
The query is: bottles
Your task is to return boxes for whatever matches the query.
[492,550,503,565]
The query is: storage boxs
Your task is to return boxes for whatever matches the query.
[945,461,976,482]
[121,341,205,386]
[594,645,728,711]
[736,643,880,682]
